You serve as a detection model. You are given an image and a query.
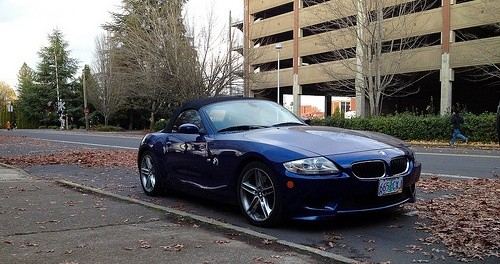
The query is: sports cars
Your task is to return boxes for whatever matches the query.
[138,97,421,227]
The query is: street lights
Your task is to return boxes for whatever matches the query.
[274,43,283,103]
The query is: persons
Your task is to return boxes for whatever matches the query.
[6,121,11,131]
[446,109,468,147]
